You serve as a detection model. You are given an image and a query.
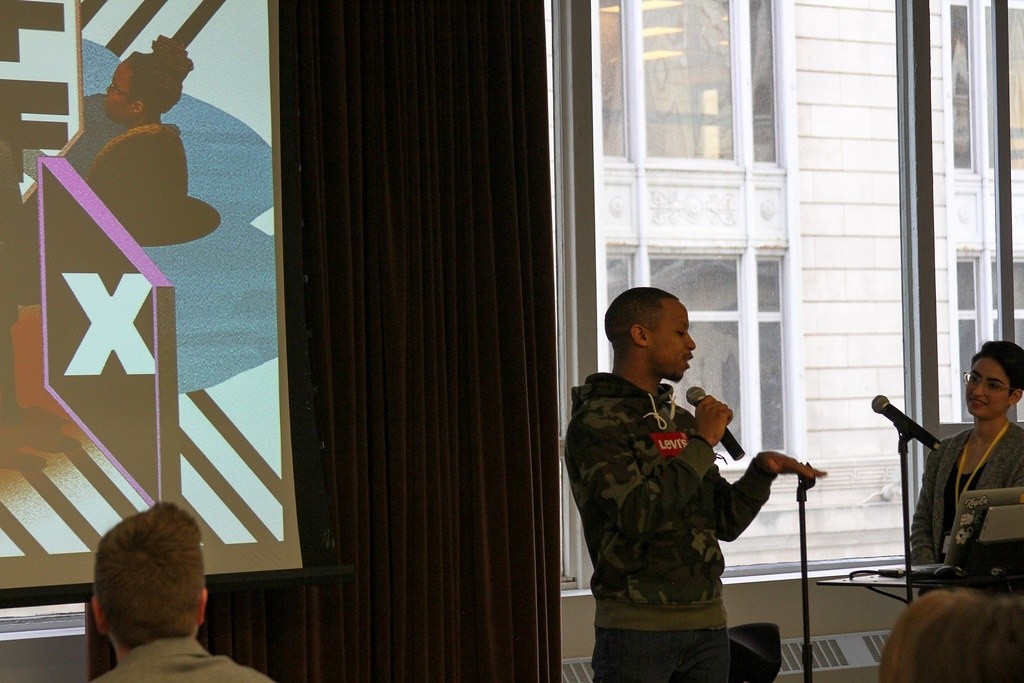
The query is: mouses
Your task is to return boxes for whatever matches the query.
[933,565,967,579]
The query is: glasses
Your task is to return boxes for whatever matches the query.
[962,372,1016,395]
[109,80,145,104]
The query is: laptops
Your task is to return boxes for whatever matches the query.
[878,486,1024,576]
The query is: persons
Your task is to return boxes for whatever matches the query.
[564,288,828,683]
[877,588,1024,683]
[89,53,189,233]
[911,341,1024,598]
[89,502,286,682]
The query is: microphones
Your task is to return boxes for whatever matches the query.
[872,395,941,451]
[686,387,745,461]
